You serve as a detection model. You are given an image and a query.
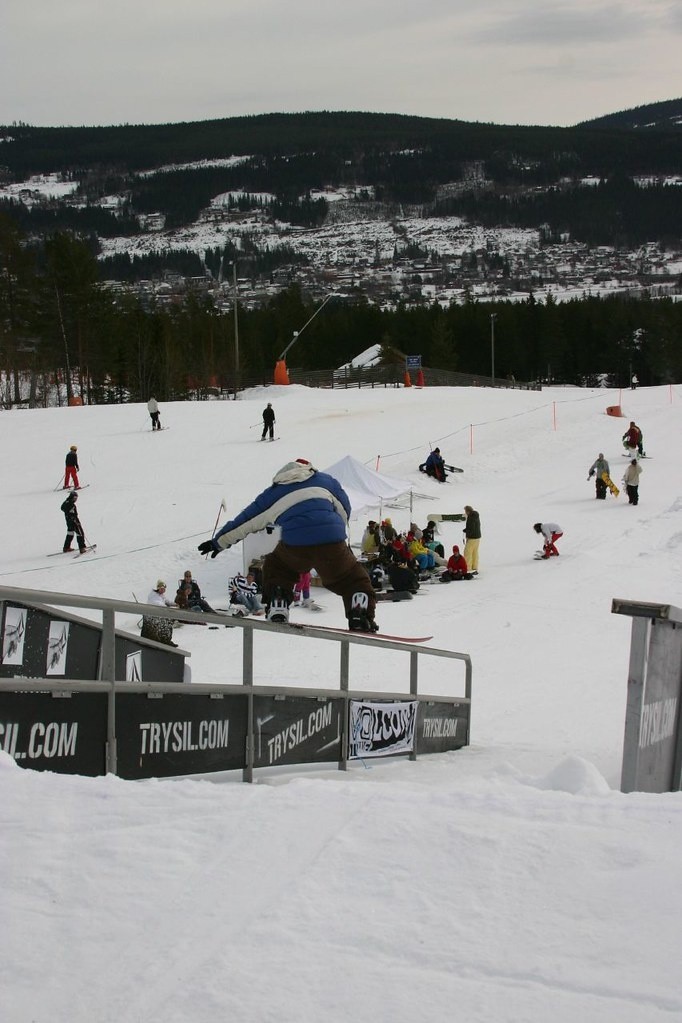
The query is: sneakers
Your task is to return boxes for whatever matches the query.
[265,600,289,623]
[348,615,377,634]
[79,548,87,554]
[64,547,74,552]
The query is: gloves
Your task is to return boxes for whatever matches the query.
[198,540,223,559]
[158,411,161,414]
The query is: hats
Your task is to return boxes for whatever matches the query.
[295,459,308,464]
[156,579,167,589]
[382,520,386,525]
[268,402,271,405]
[385,518,391,524]
[415,530,423,539]
[433,447,440,452]
[453,545,458,553]
[368,520,376,525]
[631,459,637,465]
[70,445,78,450]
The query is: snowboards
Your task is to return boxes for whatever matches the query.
[602,472,620,498]
[427,513,468,522]
[622,454,652,459]
[243,616,433,642]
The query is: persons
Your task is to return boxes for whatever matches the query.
[147,393,162,431]
[198,458,379,635]
[624,459,643,505]
[463,506,481,571]
[261,403,276,442]
[589,453,610,500]
[426,447,448,483]
[141,518,474,649]
[534,523,563,559]
[623,421,643,463]
[631,374,639,390]
[64,446,82,489]
[61,491,91,554]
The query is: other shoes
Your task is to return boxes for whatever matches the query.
[74,487,81,490]
[550,553,559,556]
[261,437,266,441]
[270,437,275,440]
[540,555,550,559]
[63,486,70,489]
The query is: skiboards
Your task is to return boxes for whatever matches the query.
[148,426,169,432]
[256,437,280,443]
[56,484,90,493]
[45,544,97,560]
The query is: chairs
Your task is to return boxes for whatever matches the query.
[178,579,206,600]
